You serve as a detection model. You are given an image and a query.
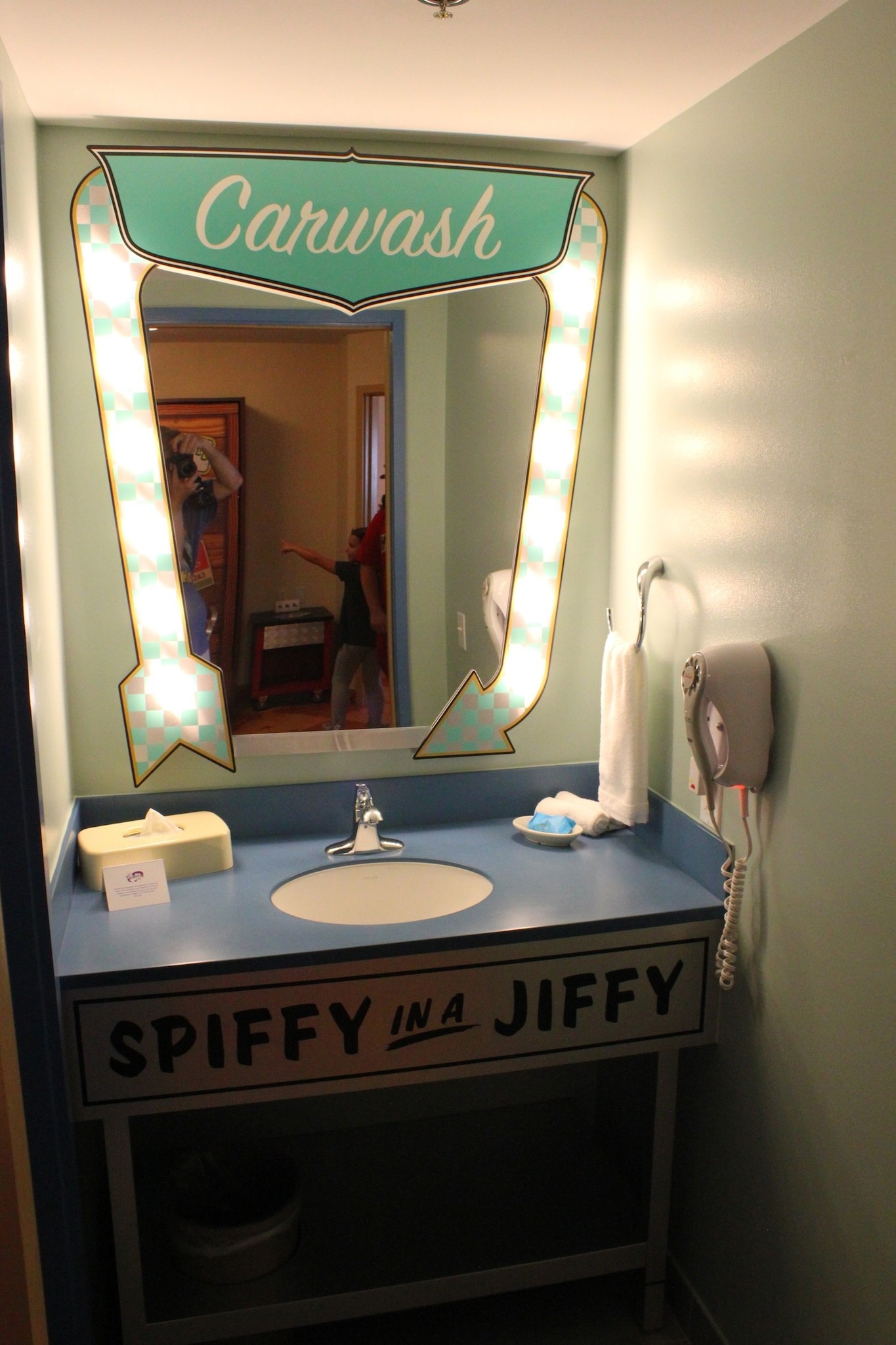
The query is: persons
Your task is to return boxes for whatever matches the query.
[159,424,245,665]
[280,528,385,729]
[356,506,388,679]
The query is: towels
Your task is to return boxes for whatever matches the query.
[597,632,653,829]
[554,789,622,831]
[533,797,609,836]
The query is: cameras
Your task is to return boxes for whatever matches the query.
[169,452,196,480]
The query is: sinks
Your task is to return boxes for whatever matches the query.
[268,857,496,925]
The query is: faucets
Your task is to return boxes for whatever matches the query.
[323,781,405,856]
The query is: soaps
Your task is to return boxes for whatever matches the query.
[526,812,579,837]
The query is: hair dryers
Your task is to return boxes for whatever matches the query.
[681,649,744,819]
[480,568,514,658]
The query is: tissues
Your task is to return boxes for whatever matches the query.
[75,807,235,892]
